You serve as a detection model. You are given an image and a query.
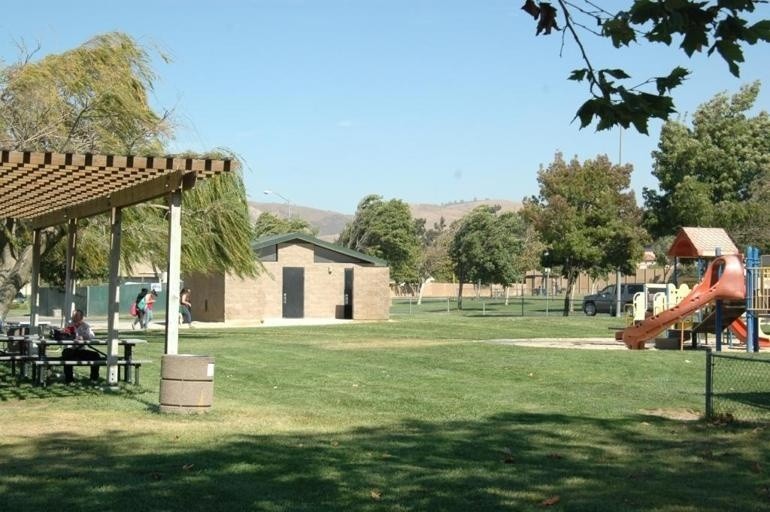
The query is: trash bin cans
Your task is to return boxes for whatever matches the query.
[52,309,61,319]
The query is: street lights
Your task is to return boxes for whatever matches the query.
[264,190,291,222]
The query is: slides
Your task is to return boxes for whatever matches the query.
[728,319,770,347]
[623,255,745,350]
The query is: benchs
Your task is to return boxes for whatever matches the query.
[0,321,153,388]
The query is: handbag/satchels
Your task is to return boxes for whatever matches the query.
[131,302,137,317]
[50,329,76,339]
[180,305,188,313]
[138,298,145,310]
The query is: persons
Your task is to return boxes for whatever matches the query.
[138,289,157,328]
[63,311,102,382]
[132,289,147,330]
[180,289,192,328]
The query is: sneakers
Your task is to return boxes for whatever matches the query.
[68,382,76,386]
[91,377,100,384]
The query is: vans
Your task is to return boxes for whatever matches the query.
[583,283,666,316]
